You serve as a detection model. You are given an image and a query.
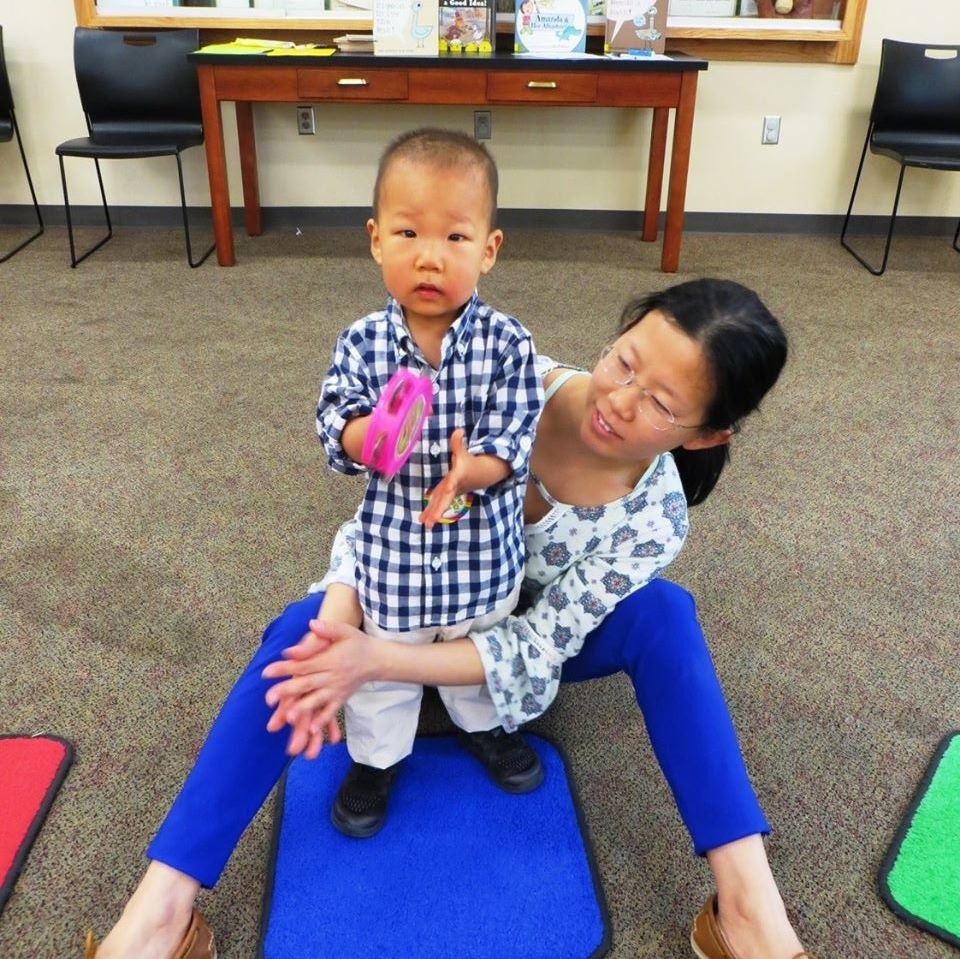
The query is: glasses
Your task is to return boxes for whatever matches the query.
[602,345,709,433]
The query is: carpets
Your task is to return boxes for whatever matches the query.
[260,731,612,959]
[880,731,960,944]
[0,734,73,911]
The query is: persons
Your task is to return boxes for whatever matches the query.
[82,276,818,959]
[314,126,546,836]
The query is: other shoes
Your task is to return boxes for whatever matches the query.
[691,891,807,959]
[85,909,218,959]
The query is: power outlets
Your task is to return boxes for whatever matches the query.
[762,116,781,145]
[297,106,315,134]
[474,111,491,139]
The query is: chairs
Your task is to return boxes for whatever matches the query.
[56,27,215,268]
[0,26,44,263]
[841,39,960,275]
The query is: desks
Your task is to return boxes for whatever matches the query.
[189,44,708,272]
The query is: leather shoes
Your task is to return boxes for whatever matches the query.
[458,721,544,794]
[331,759,398,836]
